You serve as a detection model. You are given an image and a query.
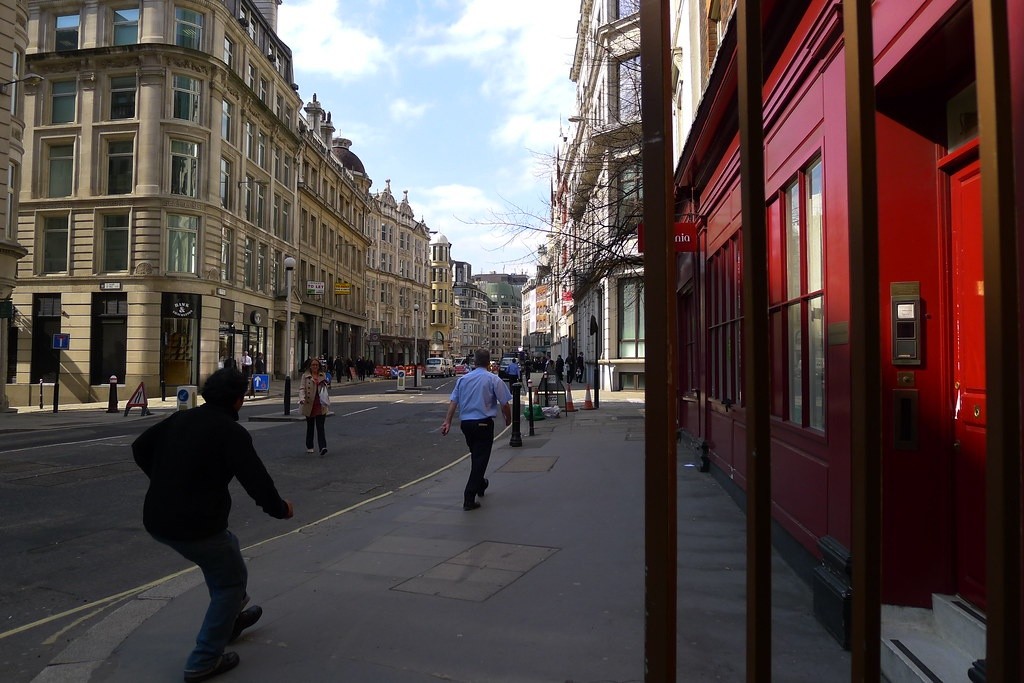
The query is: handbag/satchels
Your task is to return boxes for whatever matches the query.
[320,387,330,407]
[565,364,570,371]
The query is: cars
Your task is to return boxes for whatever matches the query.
[424,358,449,378]
[445,359,456,377]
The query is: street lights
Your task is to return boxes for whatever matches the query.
[284,257,296,415]
[414,304,419,387]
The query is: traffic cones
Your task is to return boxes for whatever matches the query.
[561,384,578,412]
[580,384,597,410]
[534,388,539,404]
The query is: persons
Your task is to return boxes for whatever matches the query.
[240,350,252,379]
[132,364,293,682]
[535,354,555,373]
[441,347,513,511]
[556,354,565,380]
[335,354,342,383]
[329,356,335,378]
[565,352,577,384]
[319,355,327,370]
[224,352,238,370]
[505,358,520,395]
[576,352,585,383]
[524,357,534,386]
[345,353,374,382]
[298,358,328,455]
[254,352,265,373]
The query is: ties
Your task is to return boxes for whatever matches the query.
[245,356,246,363]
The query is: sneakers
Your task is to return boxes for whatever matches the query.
[184,652,239,681]
[231,605,262,640]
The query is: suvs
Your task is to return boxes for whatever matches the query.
[497,358,524,382]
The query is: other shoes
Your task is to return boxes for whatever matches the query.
[478,479,488,496]
[463,502,479,511]
[320,448,327,455]
[307,448,314,453]
